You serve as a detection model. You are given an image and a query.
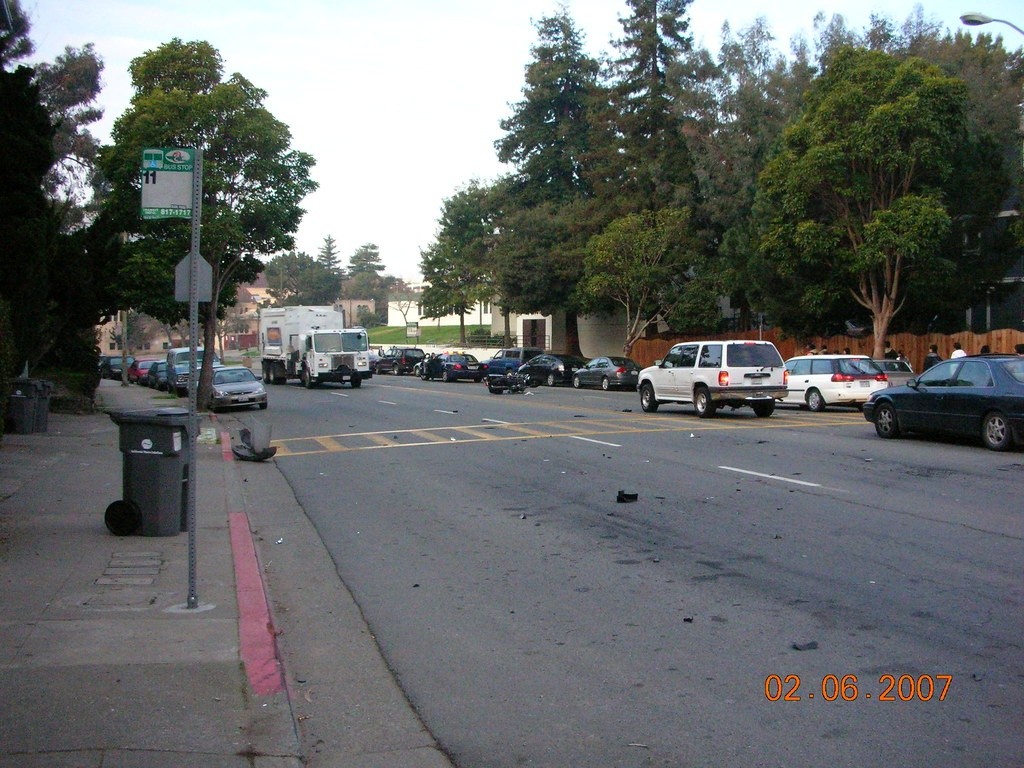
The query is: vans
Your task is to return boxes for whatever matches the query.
[165,345,223,398]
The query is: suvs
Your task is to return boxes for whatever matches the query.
[480,346,547,381]
[635,339,790,419]
[372,346,426,377]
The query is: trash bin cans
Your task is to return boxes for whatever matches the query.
[9,377,53,435]
[103,407,202,537]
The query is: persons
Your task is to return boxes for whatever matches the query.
[896,350,912,369]
[834,350,839,354]
[369,347,373,355]
[1014,344,1024,357]
[980,345,990,354]
[379,346,384,356]
[806,343,817,355]
[818,344,830,355]
[843,348,851,354]
[923,344,942,372]
[422,352,437,380]
[884,341,898,360]
[951,342,967,374]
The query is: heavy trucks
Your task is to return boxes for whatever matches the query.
[258,304,373,389]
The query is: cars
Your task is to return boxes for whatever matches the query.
[571,355,643,391]
[412,352,488,384]
[861,352,1024,453]
[207,366,268,415]
[516,353,586,387]
[97,355,226,397]
[873,358,918,388]
[773,353,890,412]
